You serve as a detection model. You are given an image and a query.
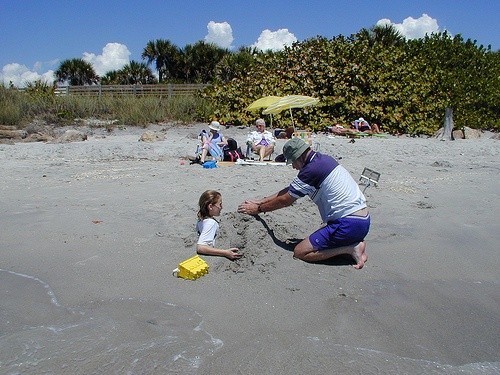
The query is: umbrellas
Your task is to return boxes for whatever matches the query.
[262,94,319,132]
[244,94,282,131]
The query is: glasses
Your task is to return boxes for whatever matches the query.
[213,202,222,206]
[256,125,262,128]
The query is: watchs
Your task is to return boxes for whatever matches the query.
[258,205,263,212]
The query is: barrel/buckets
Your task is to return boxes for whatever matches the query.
[173,254,209,278]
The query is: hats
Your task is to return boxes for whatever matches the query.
[208,121,220,131]
[283,137,311,166]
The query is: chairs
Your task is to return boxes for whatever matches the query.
[328,126,369,139]
[246,146,272,162]
[198,151,223,163]
[354,119,386,137]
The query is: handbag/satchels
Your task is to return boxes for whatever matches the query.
[223,146,244,162]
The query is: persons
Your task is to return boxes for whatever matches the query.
[329,117,382,135]
[245,119,276,163]
[195,190,243,262]
[189,121,228,165]
[237,137,370,269]
